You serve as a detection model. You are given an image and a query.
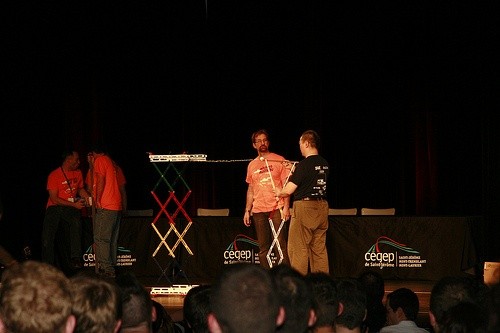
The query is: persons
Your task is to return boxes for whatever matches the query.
[271,130,334,275]
[90,142,124,281]
[360,296,386,333]
[184,284,209,333]
[359,269,384,303]
[40,150,91,279]
[379,288,429,332]
[304,271,343,332]
[485,282,500,332]
[151,300,177,333]
[270,262,314,333]
[109,272,157,333]
[243,128,296,272]
[69,269,122,333]
[85,150,127,267]
[0,260,75,333]
[429,269,490,333]
[207,262,285,332]
[332,277,367,333]
